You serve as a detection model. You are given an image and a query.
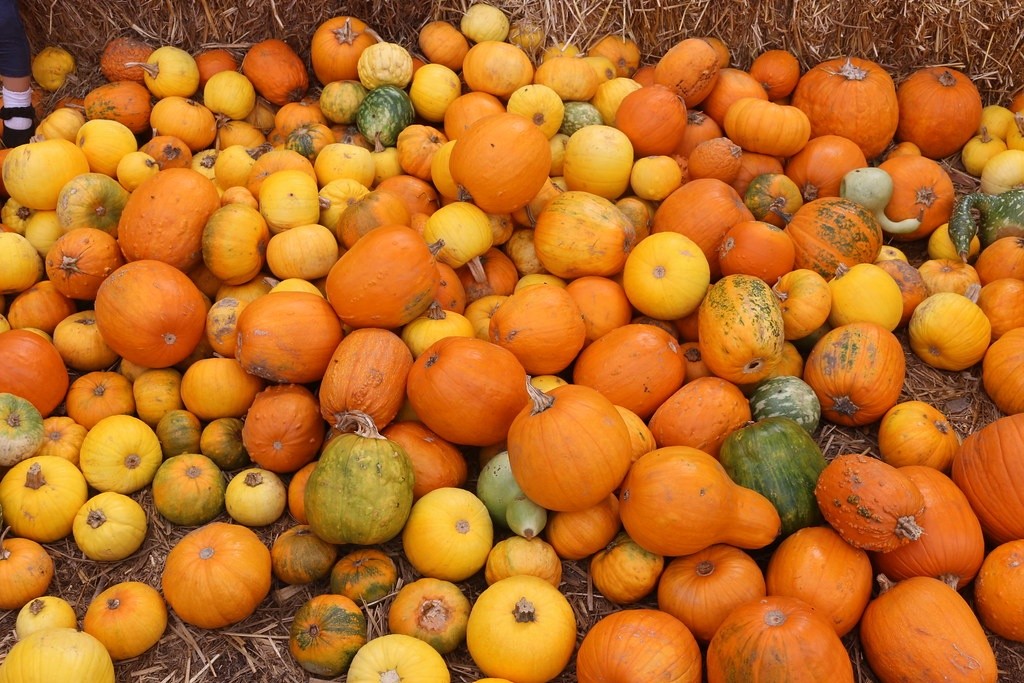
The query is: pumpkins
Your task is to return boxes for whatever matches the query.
[0,2,1024,683]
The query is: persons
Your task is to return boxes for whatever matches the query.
[0,0,38,146]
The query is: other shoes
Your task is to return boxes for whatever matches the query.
[0,104,36,148]
